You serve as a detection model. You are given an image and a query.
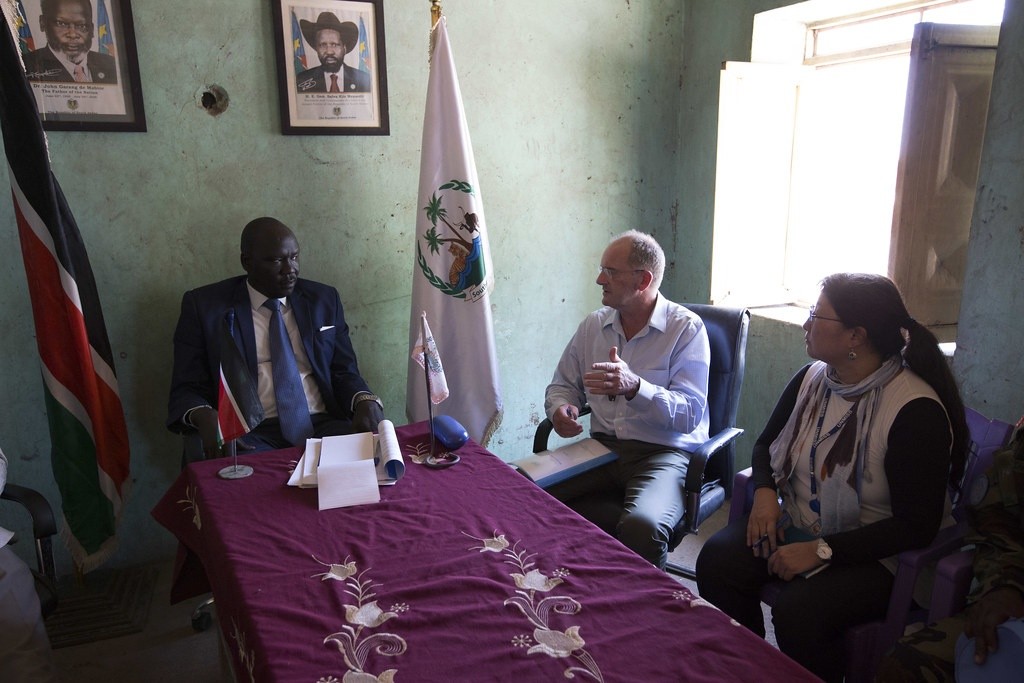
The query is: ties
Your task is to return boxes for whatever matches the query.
[262,299,315,446]
[330,74,339,93]
[74,66,88,82]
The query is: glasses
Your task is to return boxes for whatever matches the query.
[809,305,844,326]
[599,265,645,278]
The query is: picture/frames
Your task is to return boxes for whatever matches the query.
[272,0,390,135]
[0,0,147,131]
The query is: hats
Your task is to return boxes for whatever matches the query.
[300,12,359,54]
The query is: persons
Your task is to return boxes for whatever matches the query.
[695,273,971,683]
[22,2,117,84]
[164,217,384,459]
[954,422,1023,683]
[514,229,712,573]
[294,10,371,93]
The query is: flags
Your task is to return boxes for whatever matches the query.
[215,320,265,451]
[407,14,504,450]
[0,0,132,577]
[411,313,449,406]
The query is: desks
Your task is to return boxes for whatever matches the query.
[150,420,826,683]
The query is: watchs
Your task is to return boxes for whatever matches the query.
[353,394,383,412]
[816,538,833,561]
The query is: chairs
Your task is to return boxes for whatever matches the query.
[930,545,978,628]
[532,303,755,580]
[0,485,59,619]
[727,407,1016,683]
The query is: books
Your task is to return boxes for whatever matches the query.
[798,563,830,580]
[286,418,406,511]
[507,437,621,490]
